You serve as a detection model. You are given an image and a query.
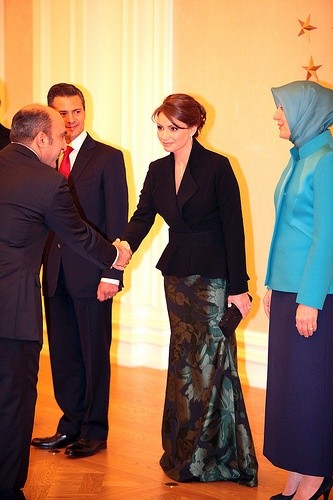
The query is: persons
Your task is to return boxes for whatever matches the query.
[112,94,258,487]
[0,104,133,500]
[0,100,11,151]
[31,83,129,457]
[263,81,333,500]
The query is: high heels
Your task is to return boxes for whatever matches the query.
[309,475,333,500]
[269,490,296,500]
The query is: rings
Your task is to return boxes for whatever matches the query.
[248,305,252,309]
[308,327,312,331]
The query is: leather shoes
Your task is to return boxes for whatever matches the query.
[64,436,107,458]
[32,432,74,449]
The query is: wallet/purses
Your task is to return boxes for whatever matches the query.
[219,293,253,340]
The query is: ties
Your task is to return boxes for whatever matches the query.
[59,146,74,182]
